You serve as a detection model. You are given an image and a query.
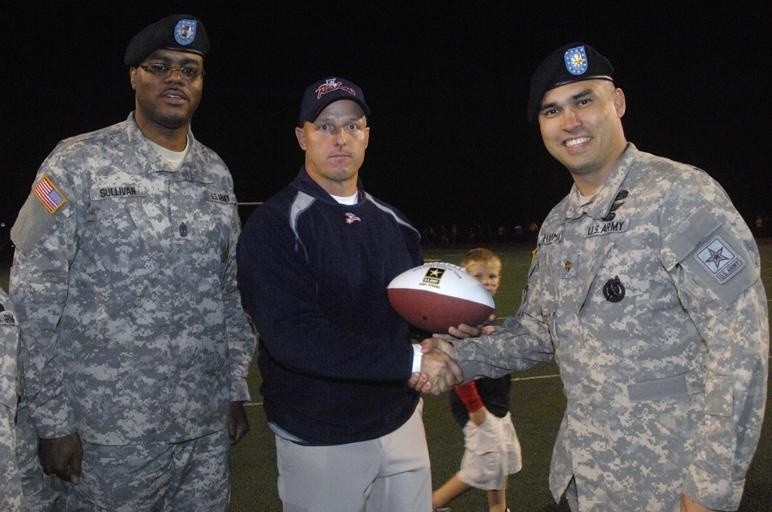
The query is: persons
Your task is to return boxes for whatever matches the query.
[9,15,256,512]
[233,76,496,511]
[432,248,523,512]
[409,43,770,512]
[0,291,36,512]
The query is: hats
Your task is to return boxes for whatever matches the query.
[524,41,617,125]
[297,74,374,130]
[123,13,210,71]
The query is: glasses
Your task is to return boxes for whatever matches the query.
[137,61,204,76]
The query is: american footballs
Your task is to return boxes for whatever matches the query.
[387,262,495,335]
